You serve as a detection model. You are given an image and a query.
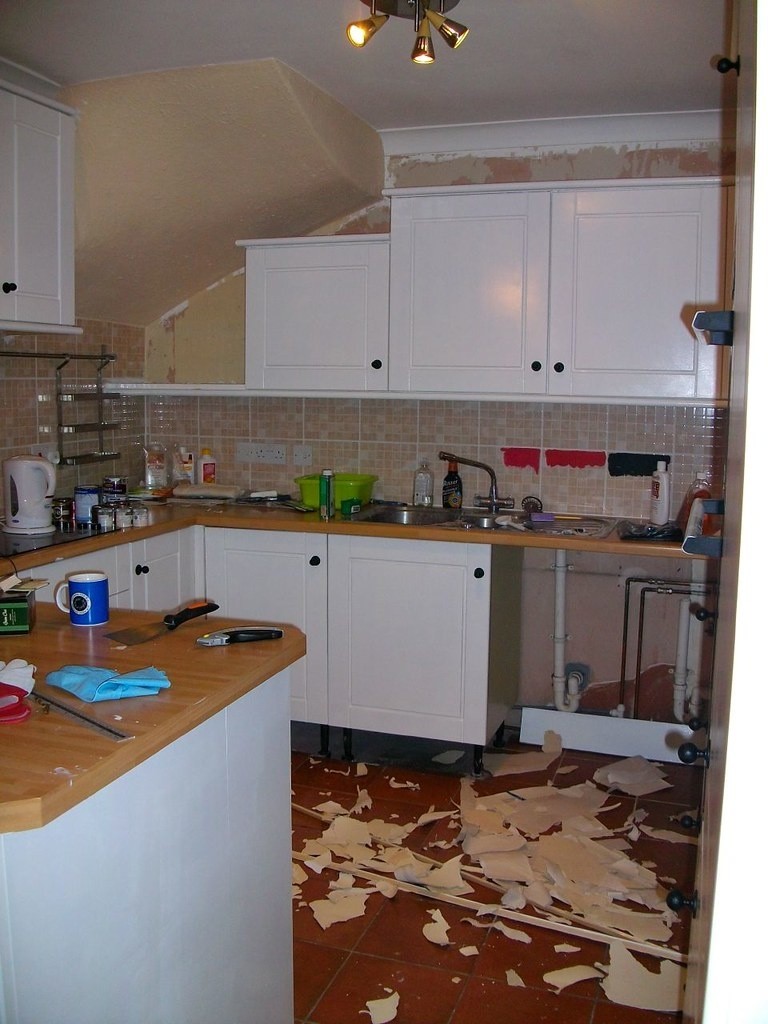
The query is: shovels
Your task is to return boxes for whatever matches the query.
[103,602,219,645]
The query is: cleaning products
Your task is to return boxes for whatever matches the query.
[442,461,463,510]
[649,458,671,526]
[412,456,434,509]
[685,471,714,536]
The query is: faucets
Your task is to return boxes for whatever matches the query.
[439,450,515,514]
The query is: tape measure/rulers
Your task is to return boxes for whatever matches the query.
[27,687,137,742]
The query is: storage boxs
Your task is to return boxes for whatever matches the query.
[295,473,379,510]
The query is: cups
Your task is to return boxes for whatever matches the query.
[93,502,126,523]
[341,496,362,513]
[52,497,74,521]
[56,573,109,627]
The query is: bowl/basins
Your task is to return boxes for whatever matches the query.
[295,472,379,510]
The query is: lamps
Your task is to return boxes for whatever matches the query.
[347,0,469,66]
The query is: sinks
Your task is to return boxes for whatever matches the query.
[338,507,457,527]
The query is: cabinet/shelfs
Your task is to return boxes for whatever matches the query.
[58,393,121,466]
[0,75,82,334]
[196,528,523,777]
[381,176,735,400]
[235,234,389,386]
[16,530,194,637]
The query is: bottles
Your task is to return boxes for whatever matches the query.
[197,448,216,485]
[686,473,712,535]
[649,461,670,526]
[145,445,167,489]
[98,501,148,527]
[173,446,194,485]
[319,470,334,519]
[412,458,434,507]
[442,462,463,509]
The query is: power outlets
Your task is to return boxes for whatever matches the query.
[293,446,313,466]
[236,443,286,466]
[29,442,57,464]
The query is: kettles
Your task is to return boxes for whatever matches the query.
[2,455,56,534]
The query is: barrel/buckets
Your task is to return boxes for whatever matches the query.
[73,485,100,523]
[102,474,128,503]
